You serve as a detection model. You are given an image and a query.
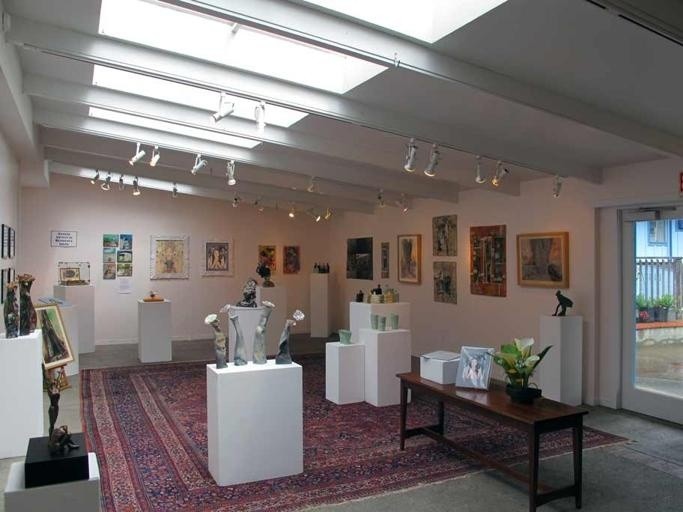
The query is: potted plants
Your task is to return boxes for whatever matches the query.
[637,289,682,326]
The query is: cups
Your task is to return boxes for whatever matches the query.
[337,328,351,344]
[369,312,398,330]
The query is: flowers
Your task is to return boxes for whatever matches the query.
[487,336,555,385]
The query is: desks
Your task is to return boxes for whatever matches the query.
[395,369,592,511]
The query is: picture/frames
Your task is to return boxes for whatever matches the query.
[1,224,15,304]
[454,345,495,393]
[396,233,422,285]
[200,238,233,277]
[516,232,570,289]
[30,303,74,369]
[150,234,190,280]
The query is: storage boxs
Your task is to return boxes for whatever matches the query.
[420,351,461,384]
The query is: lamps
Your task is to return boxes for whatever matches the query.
[86,165,331,225]
[210,85,563,201]
[123,140,413,214]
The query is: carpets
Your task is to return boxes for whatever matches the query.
[80,352,636,511]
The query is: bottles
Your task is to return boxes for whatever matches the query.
[354,283,398,304]
[312,260,328,273]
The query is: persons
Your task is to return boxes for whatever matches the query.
[218,246,228,266]
[209,248,224,269]
[44,368,63,442]
[104,264,115,278]
[49,423,81,458]
[463,353,488,388]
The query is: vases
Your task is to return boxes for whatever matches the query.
[507,381,543,402]
